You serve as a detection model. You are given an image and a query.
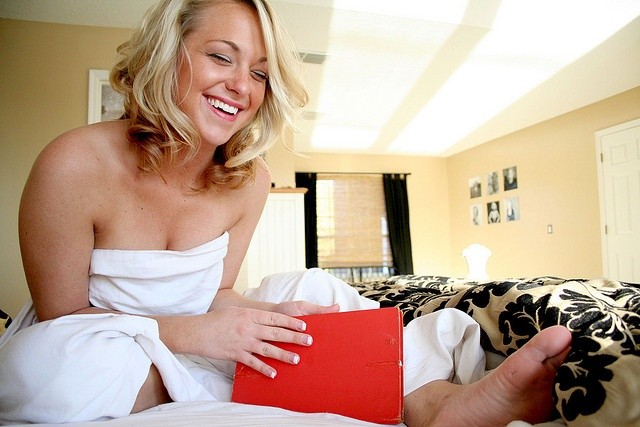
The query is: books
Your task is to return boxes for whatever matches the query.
[229,306,403,425]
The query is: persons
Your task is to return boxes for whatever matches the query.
[0,1,575,427]
